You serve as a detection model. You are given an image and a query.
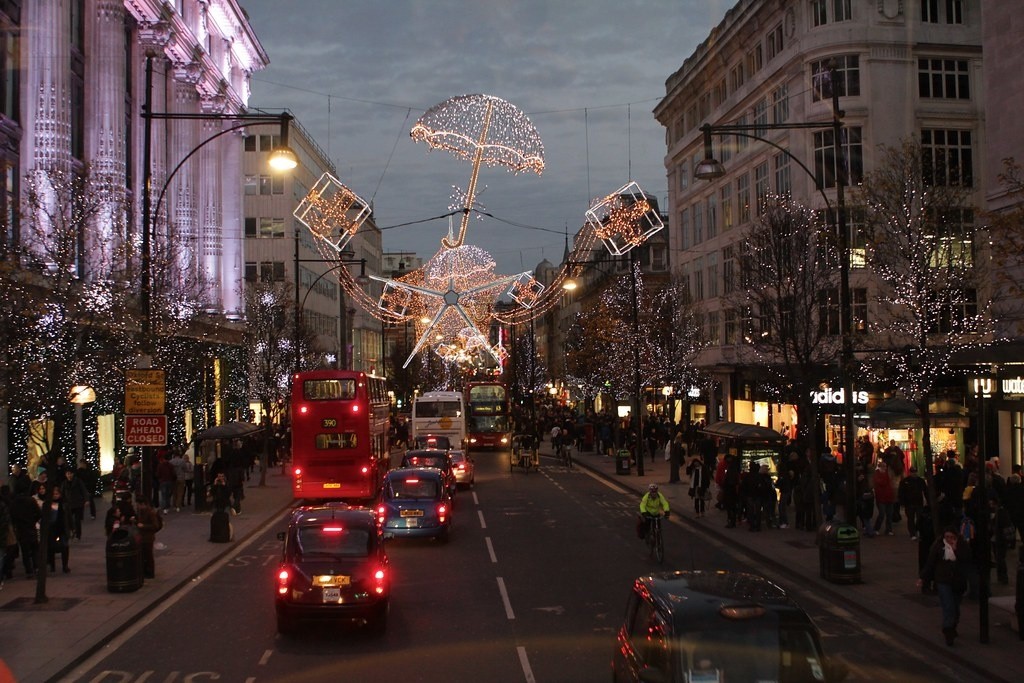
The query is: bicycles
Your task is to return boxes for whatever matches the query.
[644,514,669,563]
[563,443,573,469]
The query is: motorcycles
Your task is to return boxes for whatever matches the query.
[510,436,540,474]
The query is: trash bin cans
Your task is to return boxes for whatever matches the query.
[105,528,145,592]
[815,521,863,585]
[615,448,632,475]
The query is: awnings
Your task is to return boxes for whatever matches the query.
[871,395,968,416]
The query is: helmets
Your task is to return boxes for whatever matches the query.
[648,484,659,491]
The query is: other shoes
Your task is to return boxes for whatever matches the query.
[725,520,737,528]
[889,532,894,535]
[875,530,880,536]
[231,507,241,516]
[942,628,958,646]
[695,512,705,518]
[911,536,917,540]
[779,524,792,529]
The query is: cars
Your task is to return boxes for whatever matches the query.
[401,450,457,494]
[277,503,392,633]
[612,569,833,683]
[377,468,453,543]
[445,450,476,488]
[411,435,453,453]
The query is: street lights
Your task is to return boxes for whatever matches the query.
[295,257,369,369]
[142,111,299,568]
[380,305,431,374]
[563,261,644,477]
[696,122,854,523]
[406,327,444,399]
[485,304,536,435]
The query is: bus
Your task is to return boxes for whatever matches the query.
[291,370,397,500]
[464,382,513,449]
[412,391,469,453]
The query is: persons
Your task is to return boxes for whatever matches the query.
[916,526,969,648]
[933,424,1024,586]
[104,506,125,535]
[686,458,710,520]
[639,484,670,529]
[111,448,195,513]
[515,406,704,481]
[135,497,162,579]
[0,457,96,585]
[873,462,896,535]
[210,425,291,515]
[881,440,905,476]
[396,422,410,449]
[898,467,929,541]
[692,651,725,683]
[715,435,874,538]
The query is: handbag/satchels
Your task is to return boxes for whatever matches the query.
[701,490,712,500]
